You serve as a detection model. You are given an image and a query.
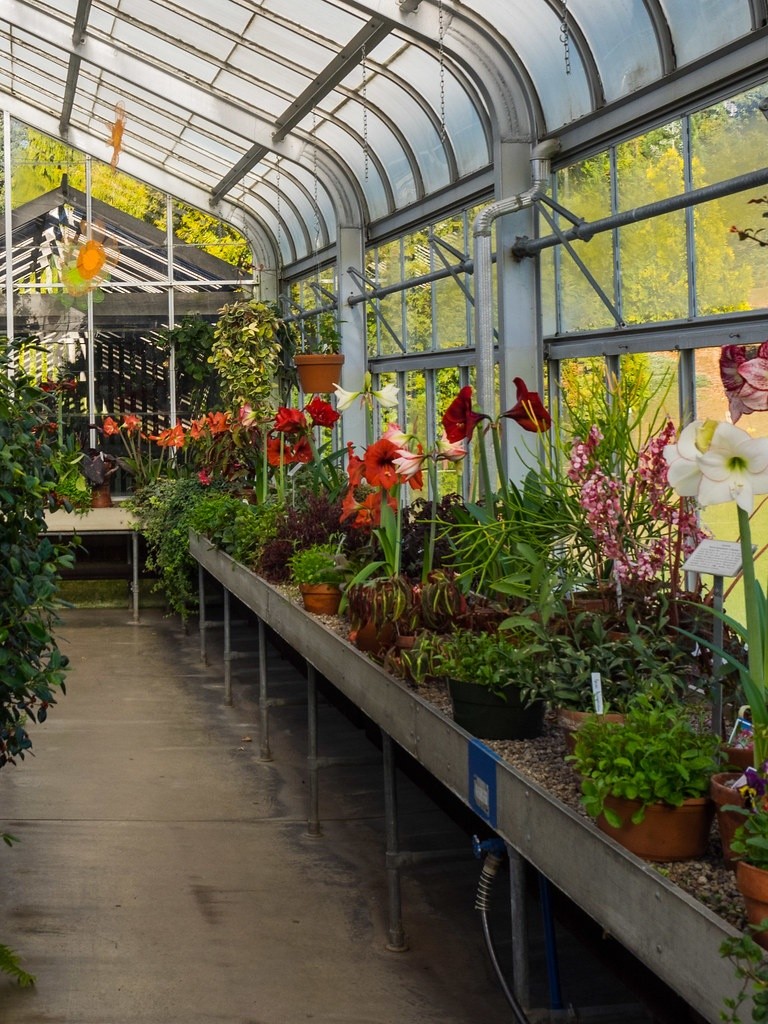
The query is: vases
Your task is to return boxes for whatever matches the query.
[597,795,708,862]
[497,682,546,740]
[395,634,447,654]
[718,745,754,772]
[446,676,497,740]
[708,772,748,862]
[561,590,617,615]
[598,619,658,644]
[735,856,768,952]
[558,707,629,795]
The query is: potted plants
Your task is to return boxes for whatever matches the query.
[60,448,137,508]
[289,307,347,393]
[284,534,355,615]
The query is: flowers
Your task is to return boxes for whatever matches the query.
[99,340,768,1024]
[105,100,127,176]
[48,217,120,310]
